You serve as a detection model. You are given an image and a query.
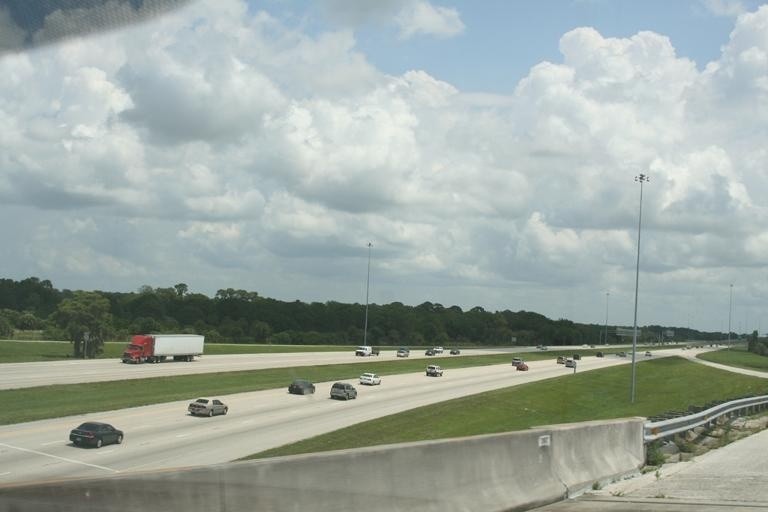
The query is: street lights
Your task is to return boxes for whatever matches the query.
[728,284,734,351]
[603,292,610,344]
[363,241,373,345]
[630,172,649,403]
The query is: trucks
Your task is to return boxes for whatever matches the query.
[355,346,380,356]
[121,334,205,364]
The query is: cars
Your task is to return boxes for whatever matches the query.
[512,357,528,371]
[397,347,410,357]
[536,344,547,350]
[616,351,653,357]
[288,379,315,395]
[557,354,581,368]
[359,373,381,385]
[188,397,229,416]
[69,422,123,448]
[426,346,460,356]
[597,351,605,356]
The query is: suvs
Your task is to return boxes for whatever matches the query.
[426,365,443,376]
[330,382,357,400]
